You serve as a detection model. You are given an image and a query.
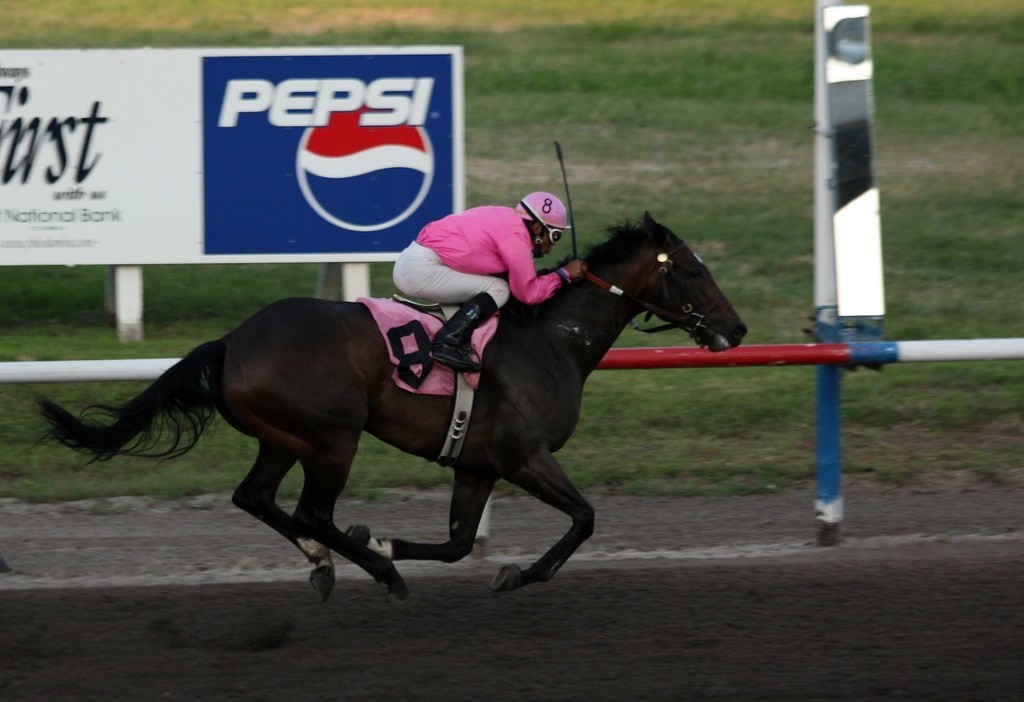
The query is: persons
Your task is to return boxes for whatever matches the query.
[393,190,589,374]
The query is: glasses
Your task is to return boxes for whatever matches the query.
[548,228,563,244]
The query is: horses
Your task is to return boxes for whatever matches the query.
[34,212,749,602]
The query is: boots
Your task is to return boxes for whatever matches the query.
[431,300,489,368]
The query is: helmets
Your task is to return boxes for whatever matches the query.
[517,192,572,229]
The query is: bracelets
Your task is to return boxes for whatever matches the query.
[556,269,569,286]
